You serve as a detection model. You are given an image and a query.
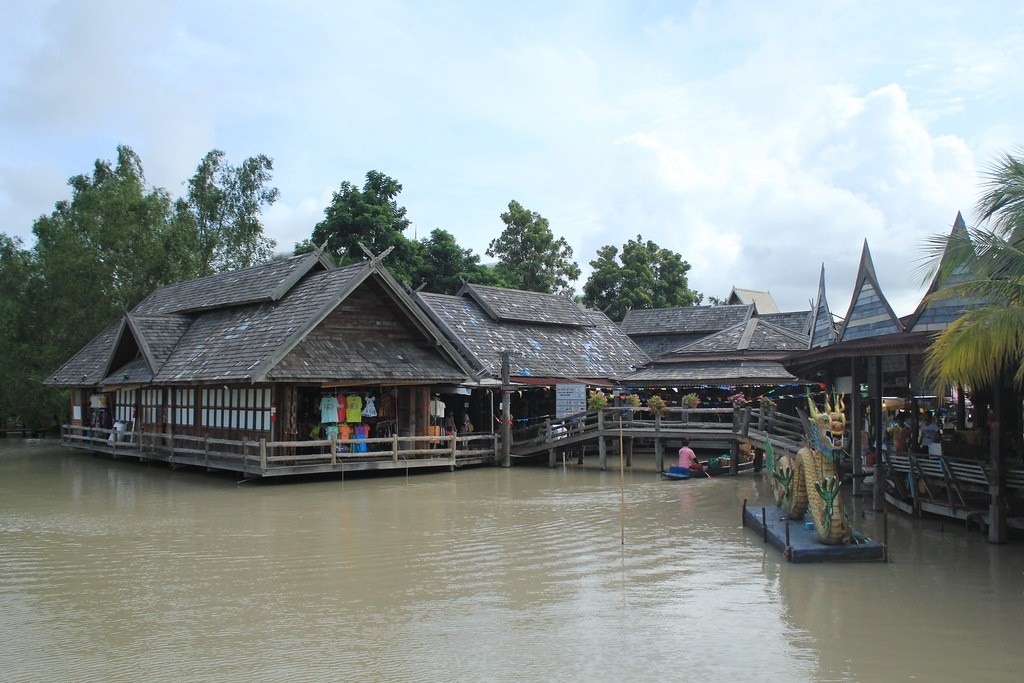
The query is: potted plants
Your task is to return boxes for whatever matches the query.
[646,395,667,418]
[681,393,701,408]
[586,390,608,409]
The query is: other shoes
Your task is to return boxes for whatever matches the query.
[465,446,467,450]
[461,446,464,450]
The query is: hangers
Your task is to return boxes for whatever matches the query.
[351,392,357,397]
[366,391,373,398]
[325,392,333,399]
[432,398,444,405]
[332,423,335,428]
[338,392,344,400]
[344,423,348,428]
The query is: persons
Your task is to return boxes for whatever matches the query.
[443,401,514,450]
[883,413,913,469]
[679,437,704,470]
[919,410,941,459]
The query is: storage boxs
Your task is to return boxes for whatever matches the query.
[719,457,730,466]
[958,429,983,446]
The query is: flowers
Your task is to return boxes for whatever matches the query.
[728,392,747,409]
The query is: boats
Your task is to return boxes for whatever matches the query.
[661,449,767,480]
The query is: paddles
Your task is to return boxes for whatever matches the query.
[691,458,715,480]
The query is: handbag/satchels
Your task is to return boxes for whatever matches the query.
[430,419,440,444]
[466,423,473,432]
[447,426,452,432]
